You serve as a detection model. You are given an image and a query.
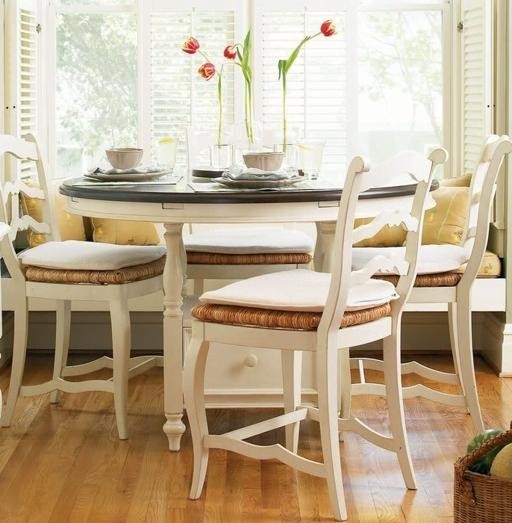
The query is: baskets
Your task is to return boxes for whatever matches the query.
[454,420,512,522]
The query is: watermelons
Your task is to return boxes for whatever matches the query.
[467,428,505,474]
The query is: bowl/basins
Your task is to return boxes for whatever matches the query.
[242,151,287,172]
[84,167,173,182]
[105,147,143,170]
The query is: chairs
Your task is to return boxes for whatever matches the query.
[1,134,177,442]
[183,144,449,520]
[343,140,511,435]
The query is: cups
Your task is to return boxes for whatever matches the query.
[210,144,232,169]
[295,138,322,181]
[153,136,179,169]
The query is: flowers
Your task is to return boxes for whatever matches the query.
[180,17,336,142]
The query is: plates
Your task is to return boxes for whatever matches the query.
[211,176,307,189]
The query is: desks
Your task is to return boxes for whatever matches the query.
[57,167,440,454]
